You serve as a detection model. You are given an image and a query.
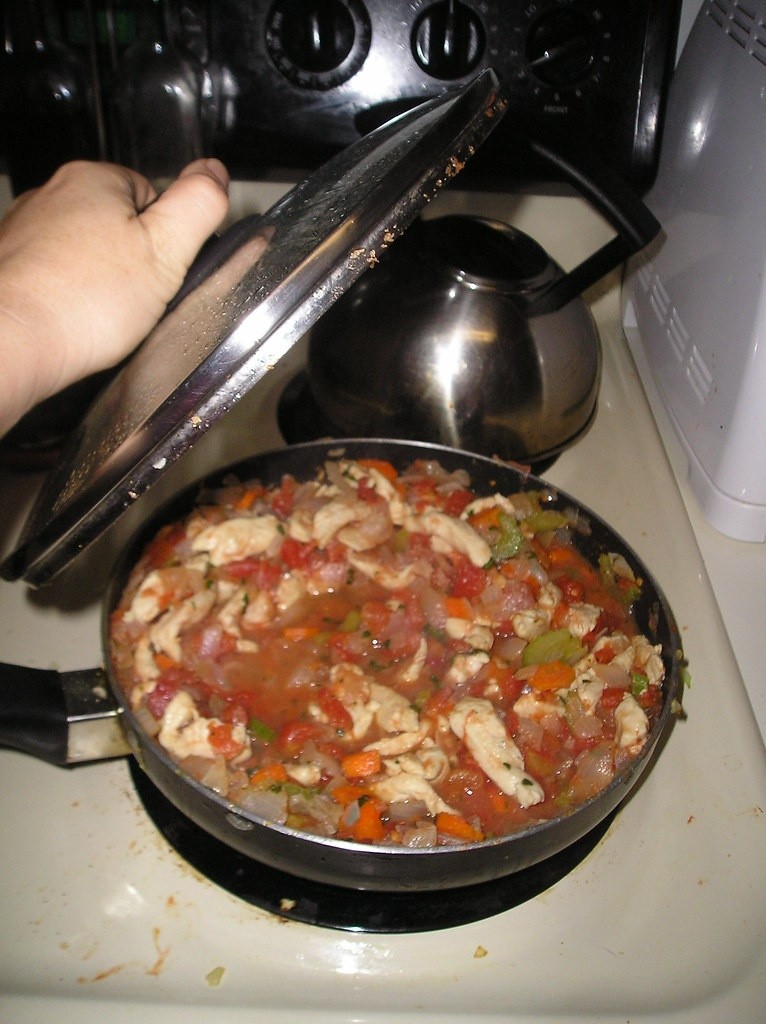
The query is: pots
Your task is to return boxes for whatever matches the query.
[0,426,684,937]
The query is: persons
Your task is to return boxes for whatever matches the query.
[0,159,231,435]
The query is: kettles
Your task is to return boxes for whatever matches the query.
[290,123,682,474]
